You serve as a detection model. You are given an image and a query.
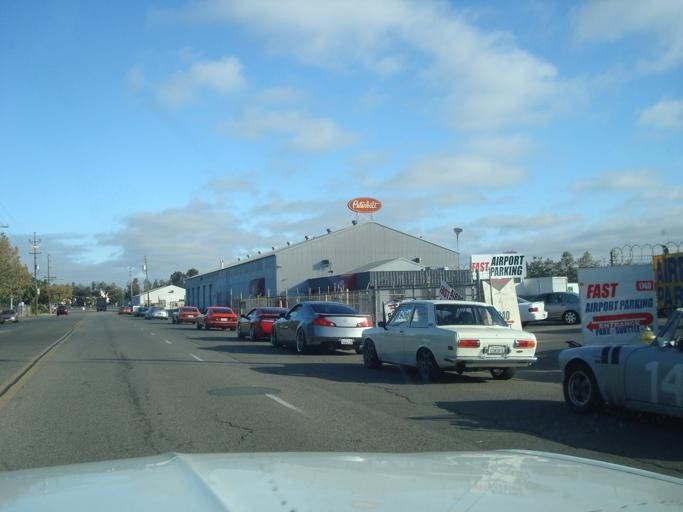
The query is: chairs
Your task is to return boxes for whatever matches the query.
[458,312,472,322]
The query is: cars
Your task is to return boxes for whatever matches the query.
[0,448,682,512]
[0,309,19,325]
[118,304,237,331]
[359,298,539,380]
[527,292,580,326]
[555,308,682,419]
[517,295,547,329]
[236,307,291,339]
[269,300,372,356]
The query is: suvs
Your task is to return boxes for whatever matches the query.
[55,304,67,317]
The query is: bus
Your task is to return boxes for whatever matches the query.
[95,297,106,312]
